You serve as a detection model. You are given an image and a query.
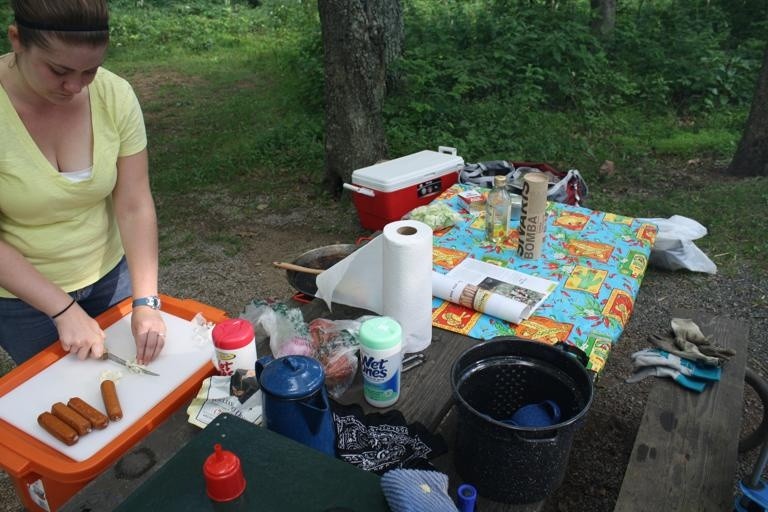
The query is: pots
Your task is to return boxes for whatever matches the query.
[286,237,375,304]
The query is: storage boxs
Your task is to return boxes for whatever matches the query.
[342,145,465,232]
[0,294,234,512]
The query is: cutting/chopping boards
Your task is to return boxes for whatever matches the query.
[0,305,215,463]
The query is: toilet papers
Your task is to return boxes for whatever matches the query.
[314,220,434,353]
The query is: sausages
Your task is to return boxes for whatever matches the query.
[101,380,123,422]
[67,398,109,429]
[52,403,93,436]
[37,411,79,446]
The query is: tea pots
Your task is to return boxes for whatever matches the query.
[255,354,338,459]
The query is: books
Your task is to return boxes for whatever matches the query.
[430,257,560,326]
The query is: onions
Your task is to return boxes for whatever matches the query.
[280,338,313,357]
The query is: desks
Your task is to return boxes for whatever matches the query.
[56,181,657,512]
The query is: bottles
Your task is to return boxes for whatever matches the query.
[358,316,402,409]
[485,174,512,243]
[211,319,259,377]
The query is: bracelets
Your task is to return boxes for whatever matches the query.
[50,299,76,320]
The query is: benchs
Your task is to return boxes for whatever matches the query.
[613,305,768,512]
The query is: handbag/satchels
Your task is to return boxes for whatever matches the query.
[458,160,589,208]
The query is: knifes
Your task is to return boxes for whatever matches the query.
[103,347,160,377]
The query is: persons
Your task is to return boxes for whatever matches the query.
[1,0,166,369]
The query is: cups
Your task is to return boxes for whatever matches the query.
[511,400,561,428]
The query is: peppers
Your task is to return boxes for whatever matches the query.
[433,202,643,347]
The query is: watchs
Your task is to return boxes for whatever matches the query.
[131,296,162,309]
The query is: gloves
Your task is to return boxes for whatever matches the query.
[624,317,738,394]
